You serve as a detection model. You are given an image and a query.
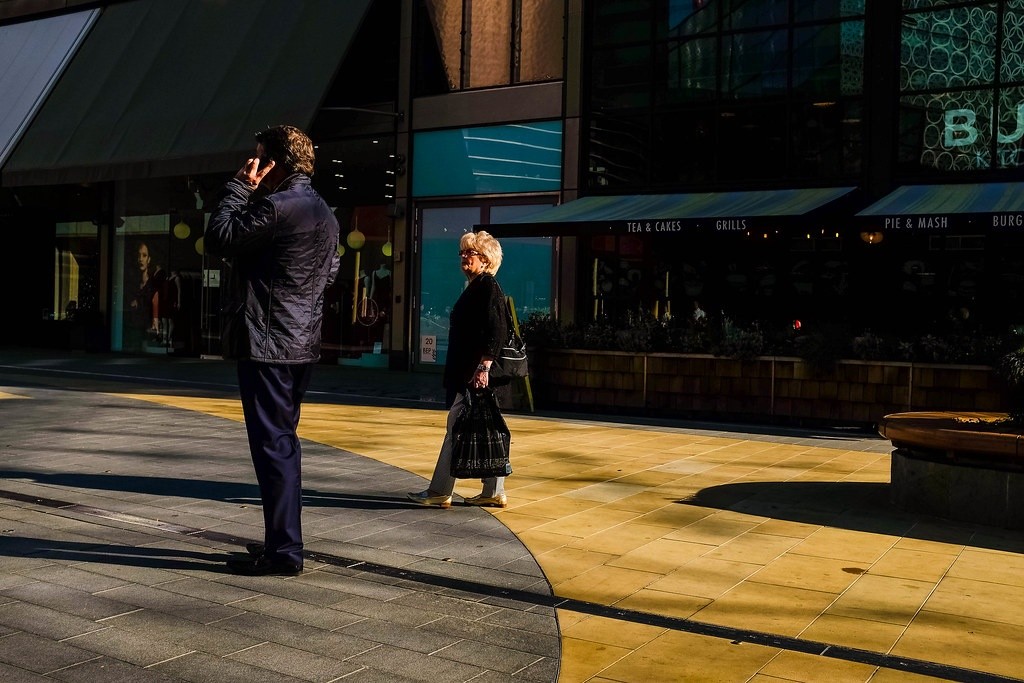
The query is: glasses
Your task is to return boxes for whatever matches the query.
[457,248,482,256]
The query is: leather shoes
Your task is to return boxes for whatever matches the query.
[245,543,271,557]
[407,492,453,509]
[225,552,304,577]
[464,493,507,508]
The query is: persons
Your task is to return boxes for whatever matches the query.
[128,241,159,330]
[407,231,513,509]
[158,270,182,346]
[369,263,391,344]
[353,268,370,340]
[203,126,339,577]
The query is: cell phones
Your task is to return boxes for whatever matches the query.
[257,147,287,175]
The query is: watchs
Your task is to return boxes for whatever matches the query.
[477,363,492,371]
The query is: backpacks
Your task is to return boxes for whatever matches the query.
[480,272,529,378]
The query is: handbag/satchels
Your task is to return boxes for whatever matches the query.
[450,386,513,478]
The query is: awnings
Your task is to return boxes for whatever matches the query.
[472,181,864,239]
[0,8,100,171]
[855,182,1024,234]
[3,2,376,187]
